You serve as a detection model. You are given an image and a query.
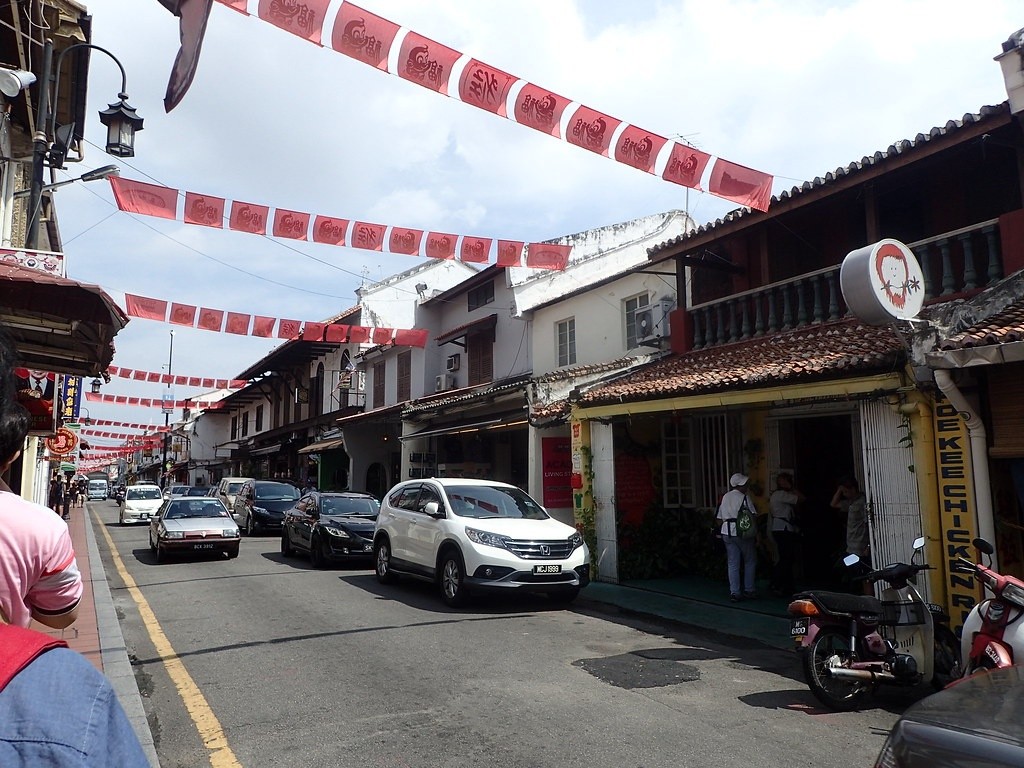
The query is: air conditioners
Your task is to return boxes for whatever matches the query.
[435,374,453,392]
[633,301,675,343]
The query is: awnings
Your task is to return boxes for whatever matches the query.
[298,439,343,454]
[164,467,179,476]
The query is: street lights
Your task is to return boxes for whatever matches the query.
[54,407,92,516]
[60,376,103,521]
[8,34,146,499]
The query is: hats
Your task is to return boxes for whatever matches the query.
[730,474,749,487]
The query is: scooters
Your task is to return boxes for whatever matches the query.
[955,537,1024,679]
[786,535,959,713]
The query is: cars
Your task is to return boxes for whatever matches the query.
[281,490,383,568]
[147,495,242,562]
[109,476,254,526]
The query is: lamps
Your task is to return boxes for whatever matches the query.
[89,376,102,393]
[80,407,91,426]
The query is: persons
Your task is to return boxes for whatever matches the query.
[18,367,53,403]
[830,476,869,594]
[716,472,762,603]
[118,483,126,495]
[768,472,806,593]
[0,395,151,768]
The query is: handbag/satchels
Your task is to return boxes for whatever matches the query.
[736,494,757,539]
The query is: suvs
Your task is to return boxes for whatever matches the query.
[372,476,592,609]
[232,479,302,537]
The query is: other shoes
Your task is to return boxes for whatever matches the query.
[744,591,760,600]
[731,593,748,602]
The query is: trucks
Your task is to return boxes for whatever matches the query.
[85,470,110,501]
